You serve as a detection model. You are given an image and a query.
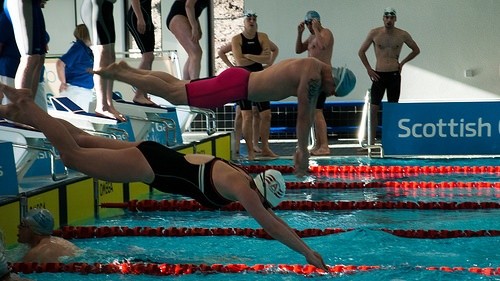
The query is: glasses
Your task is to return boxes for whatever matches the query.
[244,13,257,17]
[262,200,269,209]
[305,21,311,25]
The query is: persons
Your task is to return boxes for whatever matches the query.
[86,57,355,177]
[0,82,329,273]
[356,7,421,152]
[0,0,210,131]
[296,11,334,156]
[217,11,279,163]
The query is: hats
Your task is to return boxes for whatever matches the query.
[332,66,356,97]
[383,7,397,16]
[303,11,320,20]
[253,169,286,207]
[244,9,258,18]
